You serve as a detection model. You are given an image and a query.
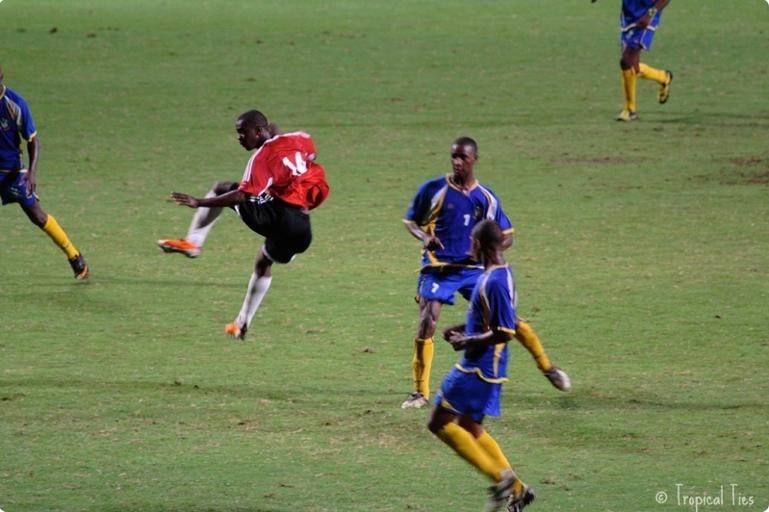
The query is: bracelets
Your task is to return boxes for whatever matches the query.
[648,5,657,18]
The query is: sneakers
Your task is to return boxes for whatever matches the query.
[68,250,89,281]
[504,483,537,512]
[615,108,640,122]
[483,478,504,512]
[222,319,249,340]
[399,390,431,410]
[155,236,205,259]
[542,365,574,393]
[657,69,674,105]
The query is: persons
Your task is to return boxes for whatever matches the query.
[402,136,571,410]
[1,70,94,281]
[427,220,535,512]
[590,0,675,123]
[156,109,328,345]
